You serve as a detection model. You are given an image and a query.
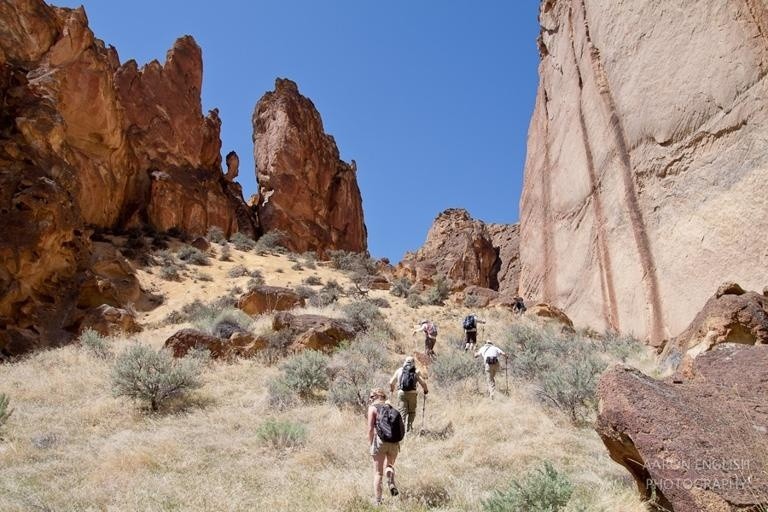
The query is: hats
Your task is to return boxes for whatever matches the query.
[372,387,388,398]
[405,356,415,365]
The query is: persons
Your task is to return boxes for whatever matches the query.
[462,312,486,353]
[413,319,437,356]
[473,339,506,398]
[366,387,405,507]
[512,295,527,316]
[389,356,429,432]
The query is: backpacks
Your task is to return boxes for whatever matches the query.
[373,402,406,443]
[428,323,438,336]
[463,315,476,330]
[399,366,417,392]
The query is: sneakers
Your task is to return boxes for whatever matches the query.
[388,486,400,499]
